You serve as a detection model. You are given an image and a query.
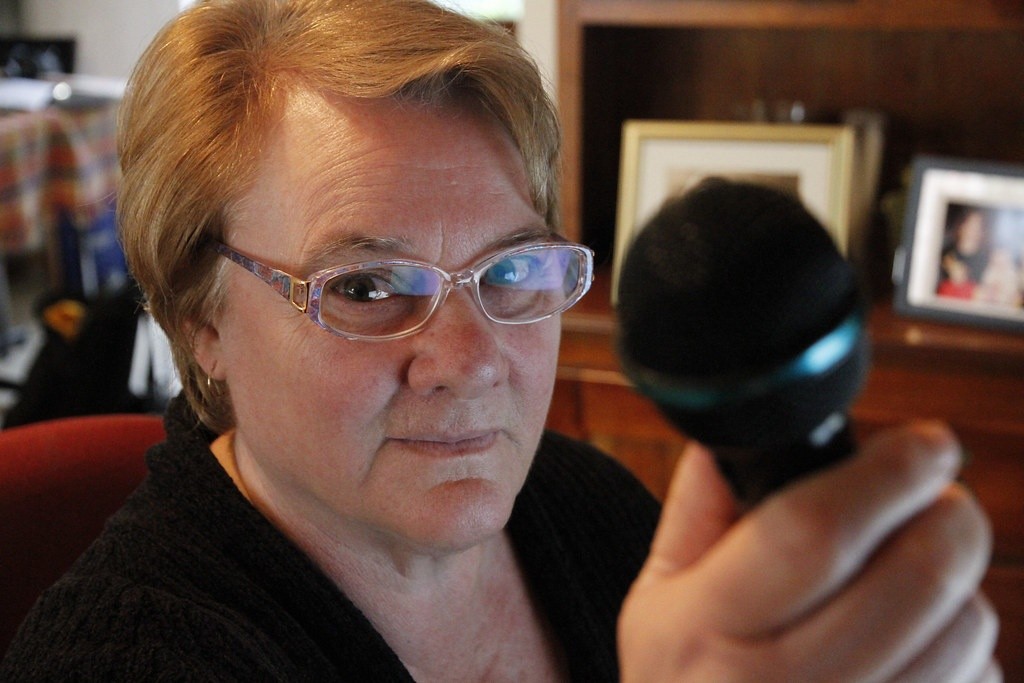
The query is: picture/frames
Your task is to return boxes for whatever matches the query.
[610,119,853,310]
[894,157,1024,338]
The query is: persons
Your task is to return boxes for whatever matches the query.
[941,210,1011,285]
[1,0,1010,683]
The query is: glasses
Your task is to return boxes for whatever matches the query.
[195,231,596,346]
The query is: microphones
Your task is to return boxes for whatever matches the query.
[610,179,868,510]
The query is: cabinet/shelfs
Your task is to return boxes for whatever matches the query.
[548,0,1024,683]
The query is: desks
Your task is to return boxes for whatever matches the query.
[0,96,119,298]
